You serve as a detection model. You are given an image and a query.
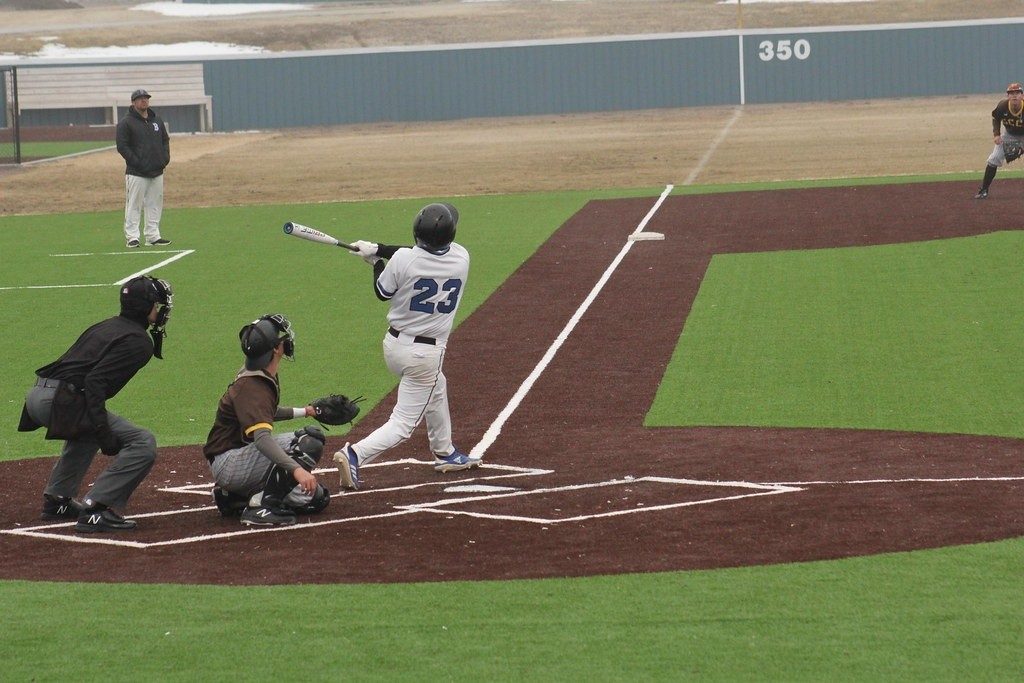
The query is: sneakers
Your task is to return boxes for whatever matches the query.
[332,441,359,490]
[212,486,238,516]
[434,445,483,474]
[41,498,82,519]
[126,241,140,247]
[241,505,295,526]
[145,238,171,245]
[76,506,136,533]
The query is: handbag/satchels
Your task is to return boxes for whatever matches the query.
[18,402,41,432]
[45,380,93,440]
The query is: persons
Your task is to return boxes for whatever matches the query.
[976,83,1024,197]
[203,314,359,524]
[116,89,173,247]
[17,275,174,532]
[333,203,483,489]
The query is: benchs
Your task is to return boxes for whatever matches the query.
[4,64,213,131]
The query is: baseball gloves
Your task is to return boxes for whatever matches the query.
[309,394,360,425]
[1002,140,1024,163]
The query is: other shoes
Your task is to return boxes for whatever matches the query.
[975,187,988,199]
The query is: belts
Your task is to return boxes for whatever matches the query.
[208,456,215,466]
[36,378,75,390]
[388,326,436,345]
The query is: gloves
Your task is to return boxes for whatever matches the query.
[364,255,380,265]
[350,240,379,258]
[99,435,122,456]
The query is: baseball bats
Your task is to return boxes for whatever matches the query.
[283,222,360,252]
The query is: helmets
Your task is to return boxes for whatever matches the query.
[241,318,279,370]
[413,202,459,249]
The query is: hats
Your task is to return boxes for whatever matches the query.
[131,90,151,99]
[120,279,161,315]
[1006,83,1022,94]
